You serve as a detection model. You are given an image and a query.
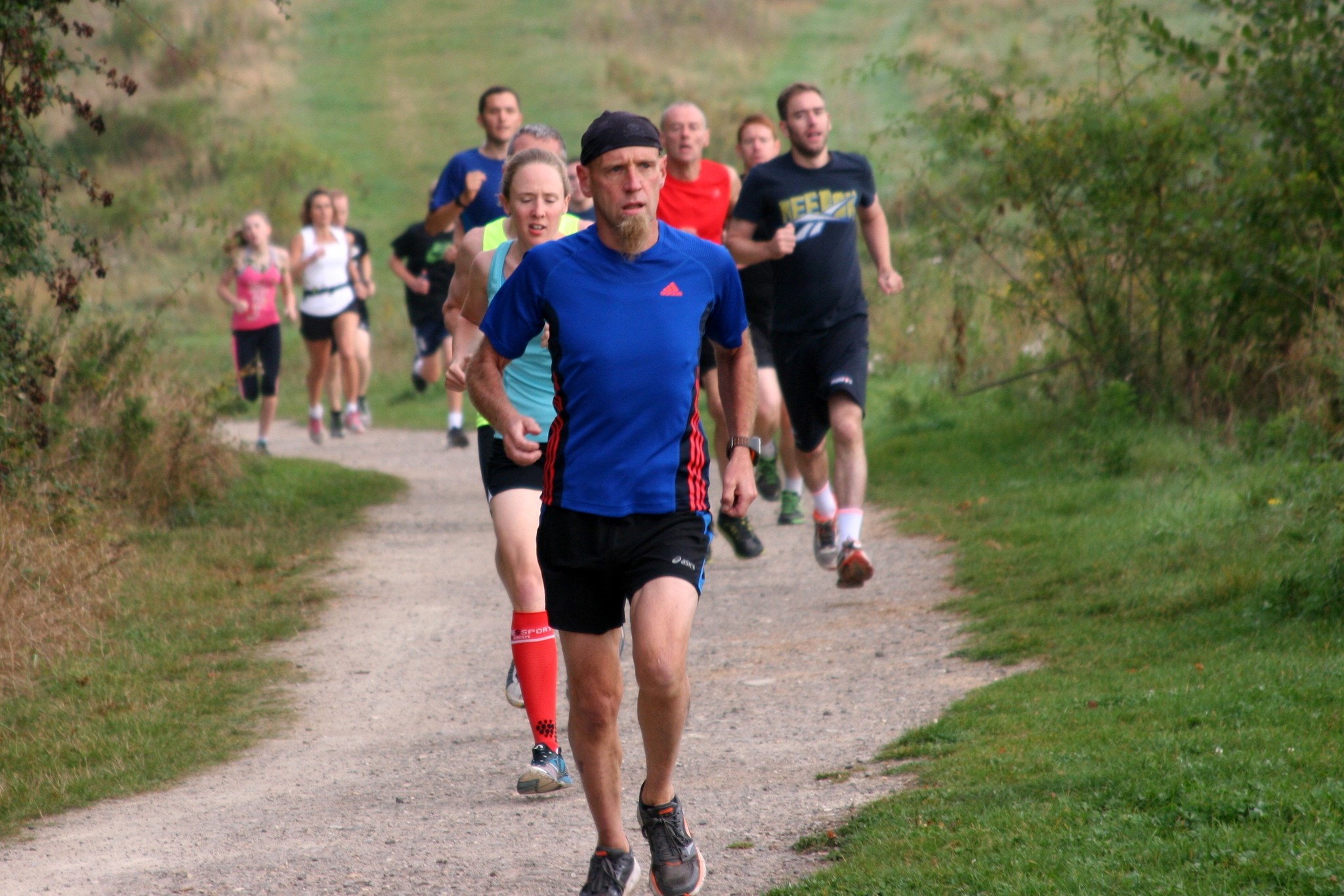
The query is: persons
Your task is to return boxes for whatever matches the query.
[444,148,572,795]
[218,84,804,708]
[727,83,904,589]
[463,112,763,896]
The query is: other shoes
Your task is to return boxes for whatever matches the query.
[449,427,468,447]
[357,395,370,423]
[344,411,364,433]
[255,441,270,456]
[309,418,326,445]
[331,412,344,437]
[411,355,427,393]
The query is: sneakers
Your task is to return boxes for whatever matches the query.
[506,659,525,709]
[717,509,766,559]
[705,547,713,563]
[579,835,641,896]
[753,442,780,501]
[836,538,873,588]
[517,743,573,795]
[814,496,840,570]
[636,779,707,896]
[778,491,804,523]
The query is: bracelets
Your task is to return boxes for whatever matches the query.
[454,194,468,209]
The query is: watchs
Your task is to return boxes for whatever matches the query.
[727,436,761,466]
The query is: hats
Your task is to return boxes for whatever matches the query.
[580,110,663,166]
